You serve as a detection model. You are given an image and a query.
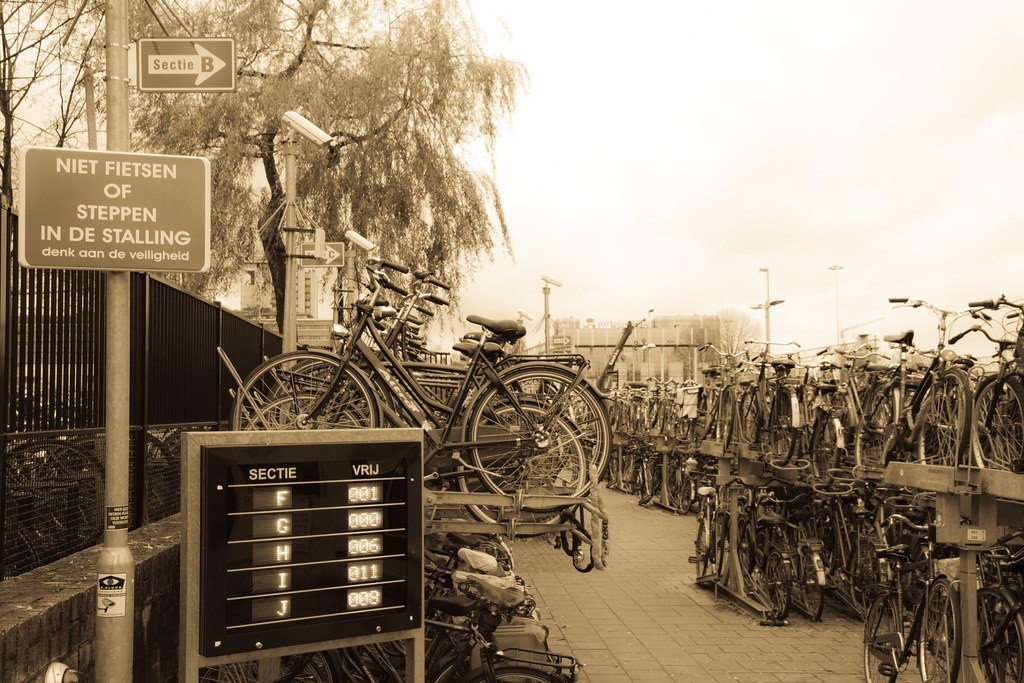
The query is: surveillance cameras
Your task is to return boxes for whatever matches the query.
[344,231,376,251]
[281,110,333,148]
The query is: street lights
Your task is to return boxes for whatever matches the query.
[829,264,845,344]
[751,267,785,354]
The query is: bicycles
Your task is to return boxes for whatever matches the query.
[605,295,1024,683]
[199,256,613,682]
[0,394,217,586]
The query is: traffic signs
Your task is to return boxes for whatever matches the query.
[137,37,237,94]
[551,335,572,347]
[300,242,345,268]
